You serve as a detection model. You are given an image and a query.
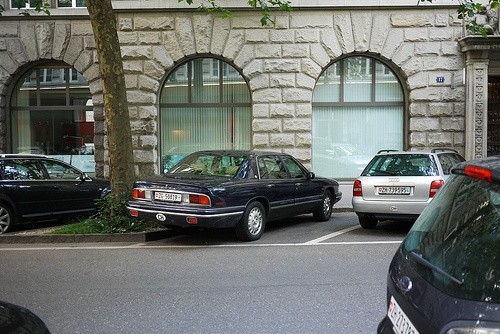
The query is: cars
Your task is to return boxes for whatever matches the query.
[124,150,343,240]
[376,155,500,334]
[0,152,110,233]
[352,148,466,227]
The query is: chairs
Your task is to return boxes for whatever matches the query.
[189,162,279,179]
[386,162,435,173]
